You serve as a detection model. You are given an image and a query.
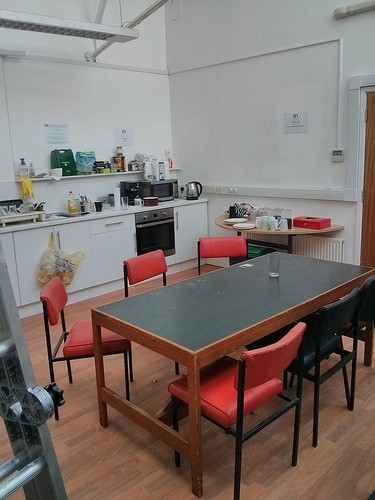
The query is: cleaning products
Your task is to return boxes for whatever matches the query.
[67,191,77,213]
[19,157,36,179]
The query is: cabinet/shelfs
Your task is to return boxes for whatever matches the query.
[0,199,209,319]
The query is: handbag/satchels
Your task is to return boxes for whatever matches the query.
[37,231,82,290]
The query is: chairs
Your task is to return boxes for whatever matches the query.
[288,276,375,408]
[198,236,249,276]
[249,287,360,448]
[169,322,306,500]
[123,249,180,376]
[40,276,133,421]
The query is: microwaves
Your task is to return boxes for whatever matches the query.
[151,178,178,202]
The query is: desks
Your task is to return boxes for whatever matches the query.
[215,215,344,254]
[91,251,375,498]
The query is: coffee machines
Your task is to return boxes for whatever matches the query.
[120,180,141,205]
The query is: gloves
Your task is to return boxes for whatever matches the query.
[17,176,35,200]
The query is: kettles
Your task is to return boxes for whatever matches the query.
[185,181,203,200]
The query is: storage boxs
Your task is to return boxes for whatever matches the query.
[293,215,331,230]
[113,156,126,172]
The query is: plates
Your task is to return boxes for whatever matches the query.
[232,224,255,229]
[224,218,247,225]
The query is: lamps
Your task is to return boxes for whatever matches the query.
[0,0,139,44]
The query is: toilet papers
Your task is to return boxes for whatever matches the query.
[114,188,120,209]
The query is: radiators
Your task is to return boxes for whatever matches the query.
[247,232,345,263]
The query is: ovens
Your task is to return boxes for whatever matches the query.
[135,207,176,257]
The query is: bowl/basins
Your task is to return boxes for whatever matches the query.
[143,197,159,206]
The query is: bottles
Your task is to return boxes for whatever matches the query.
[108,194,114,206]
[69,191,76,212]
[159,162,165,180]
[224,211,229,220]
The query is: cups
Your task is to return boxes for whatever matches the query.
[111,163,117,173]
[0,205,8,216]
[271,207,284,231]
[229,206,236,218]
[268,253,280,277]
[251,207,263,229]
[122,197,128,209]
[103,204,110,212]
[134,199,144,207]
[9,205,17,216]
[95,201,102,212]
[20,203,34,214]
[262,207,274,230]
[285,209,291,217]
[276,218,292,231]
